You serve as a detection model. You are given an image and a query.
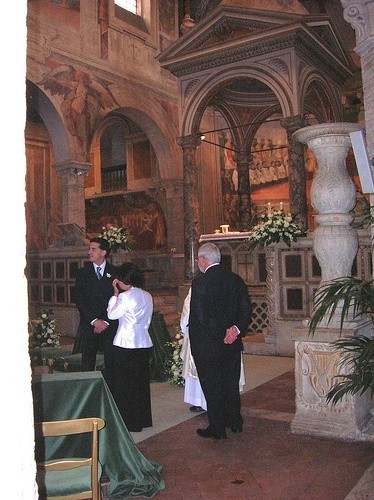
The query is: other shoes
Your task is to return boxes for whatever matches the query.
[190,406,205,412]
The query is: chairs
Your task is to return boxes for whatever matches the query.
[36,417,106,500]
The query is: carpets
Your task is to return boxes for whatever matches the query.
[98,368,374,500]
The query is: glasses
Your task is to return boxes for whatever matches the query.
[195,258,198,263]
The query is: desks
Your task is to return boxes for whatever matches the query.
[32,371,166,498]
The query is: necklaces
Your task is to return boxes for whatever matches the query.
[128,285,132,288]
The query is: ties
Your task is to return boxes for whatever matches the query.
[96,267,102,280]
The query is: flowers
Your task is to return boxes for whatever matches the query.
[30,308,61,347]
[99,225,133,252]
[163,321,185,388]
[246,210,302,250]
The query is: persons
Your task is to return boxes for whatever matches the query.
[107,262,153,431]
[72,237,119,373]
[180,243,252,438]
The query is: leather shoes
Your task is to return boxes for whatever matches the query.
[197,429,228,439]
[225,424,242,433]
